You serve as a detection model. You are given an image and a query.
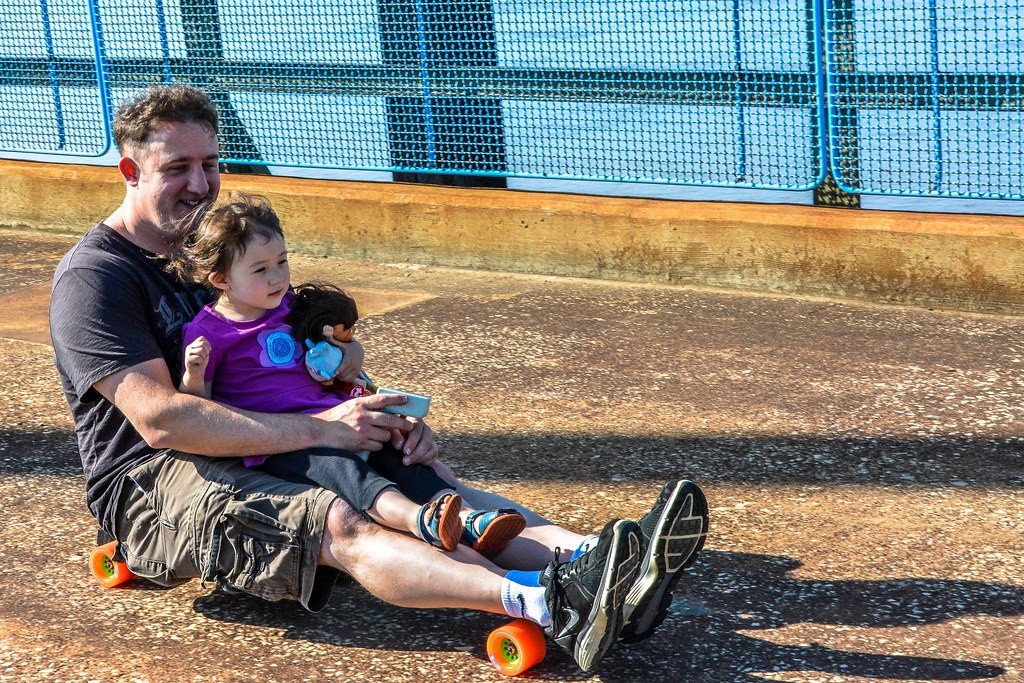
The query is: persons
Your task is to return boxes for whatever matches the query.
[150,188,527,563]
[43,84,715,675]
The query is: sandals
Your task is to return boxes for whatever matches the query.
[460,507,527,562]
[416,491,464,552]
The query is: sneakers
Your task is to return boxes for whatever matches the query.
[616,478,711,644]
[542,517,644,672]
